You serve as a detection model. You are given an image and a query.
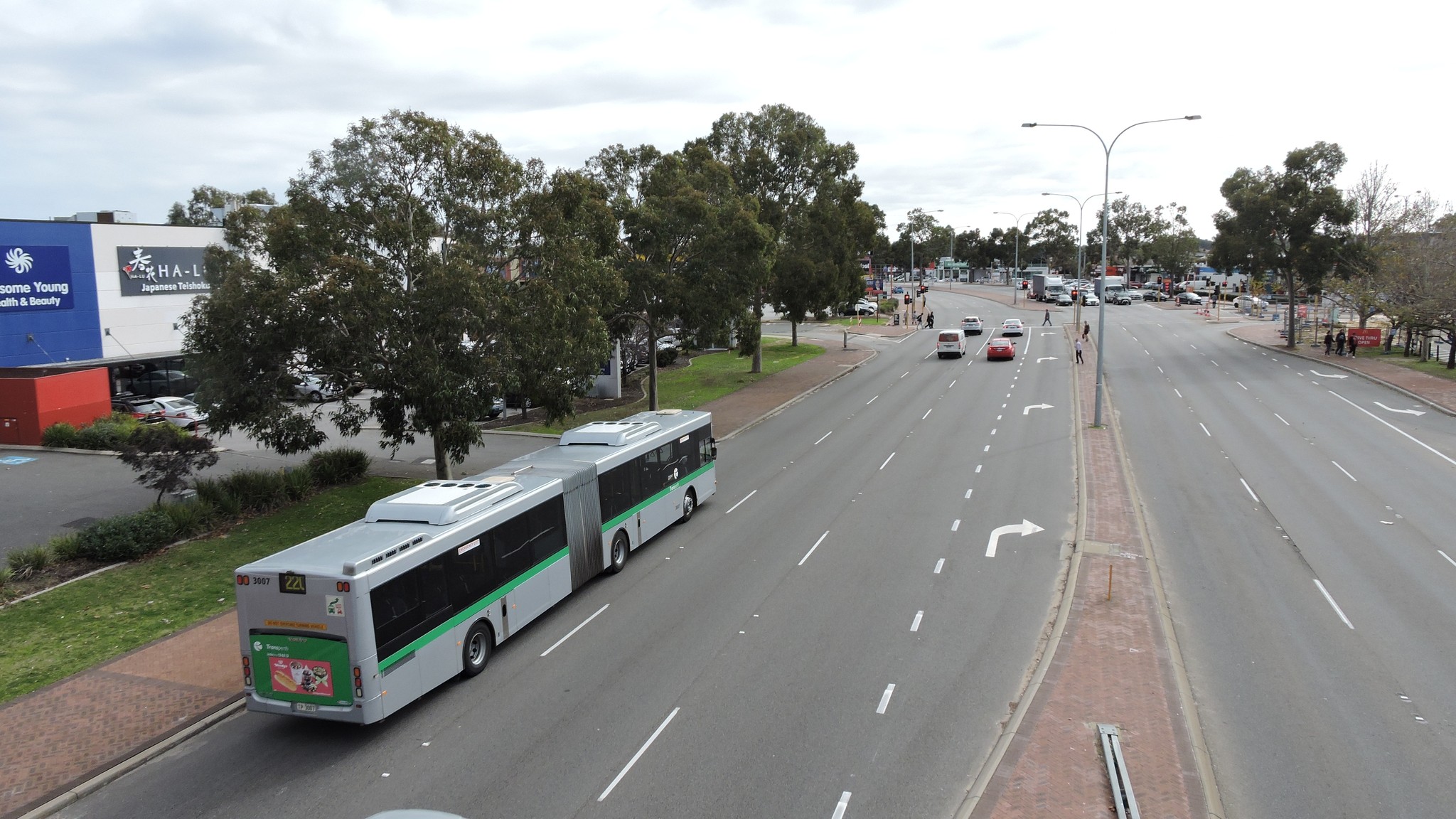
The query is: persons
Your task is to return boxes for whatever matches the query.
[1074,338,1084,364]
[1041,309,1052,326]
[1346,332,1358,359]
[923,295,926,307]
[874,280,881,290]
[912,311,917,325]
[916,313,924,329]
[925,313,931,329]
[1211,294,1216,308]
[1084,320,1090,341]
[903,310,909,325]
[931,312,935,328]
[1335,329,1347,356]
[1323,330,1335,357]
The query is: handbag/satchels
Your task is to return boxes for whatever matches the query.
[1082,333,1084,339]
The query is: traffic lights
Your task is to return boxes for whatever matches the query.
[1071,289,1078,301]
[1211,281,1215,286]
[1158,276,1162,284]
[904,294,912,305]
[1206,278,1210,286]
[916,285,929,297]
[1022,281,1028,289]
[1222,282,1227,286]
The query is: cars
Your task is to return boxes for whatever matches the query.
[892,274,958,282]
[621,327,686,373]
[937,316,1024,361]
[976,275,1207,307]
[837,294,878,318]
[892,286,904,294]
[1233,295,1269,310]
[458,364,578,422]
[111,360,370,432]
[1175,292,1202,305]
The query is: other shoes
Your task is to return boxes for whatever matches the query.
[1328,354,1331,357]
[1050,325,1052,326]
[1042,324,1044,326]
[1346,353,1349,357]
[1352,356,1355,358]
[1325,353,1326,356]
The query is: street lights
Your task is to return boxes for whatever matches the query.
[994,212,1045,305]
[911,210,944,319]
[949,226,971,290]
[1395,190,1422,237]
[1021,115,1203,428]
[1041,191,1123,331]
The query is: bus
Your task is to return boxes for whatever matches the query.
[234,409,719,725]
